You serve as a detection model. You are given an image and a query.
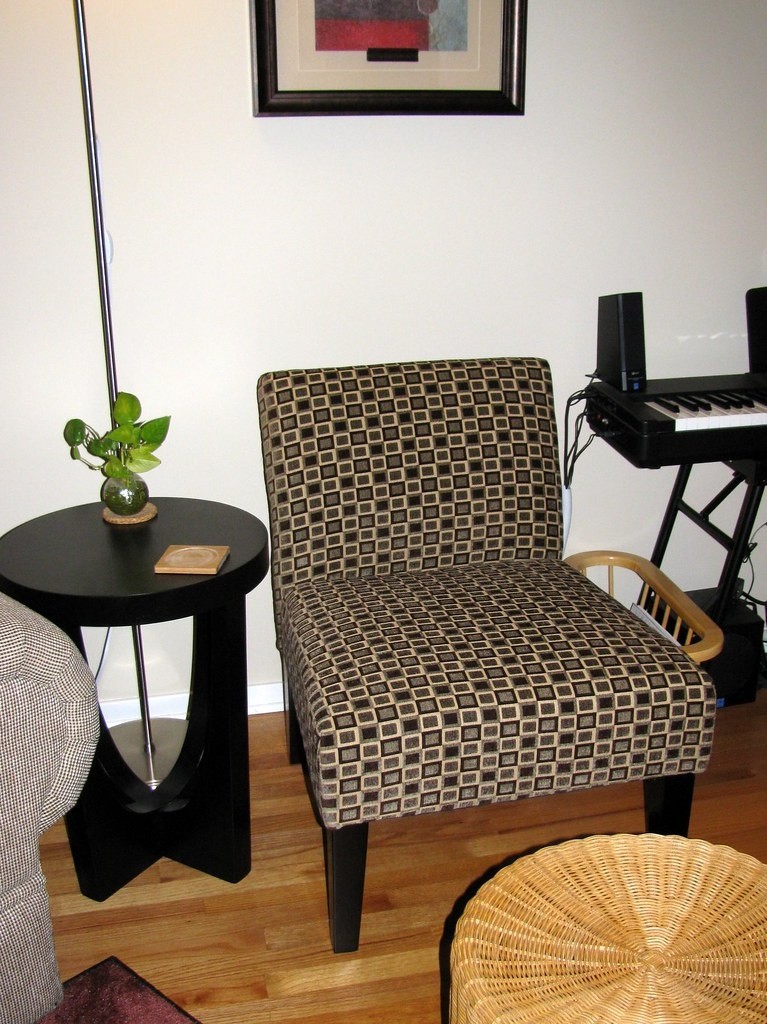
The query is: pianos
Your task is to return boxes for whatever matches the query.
[584,368,767,628]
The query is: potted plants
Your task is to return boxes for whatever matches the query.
[62,392,171,514]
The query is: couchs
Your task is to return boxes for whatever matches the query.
[0,592,102,1024]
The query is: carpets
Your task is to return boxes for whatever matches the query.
[37,955,203,1024]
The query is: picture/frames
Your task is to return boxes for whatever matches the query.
[248,1,529,118]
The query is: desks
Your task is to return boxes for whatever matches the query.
[447,833,767,1023]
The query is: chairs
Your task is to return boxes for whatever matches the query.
[254,358,715,952]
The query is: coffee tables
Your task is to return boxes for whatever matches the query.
[0,497,268,903]
